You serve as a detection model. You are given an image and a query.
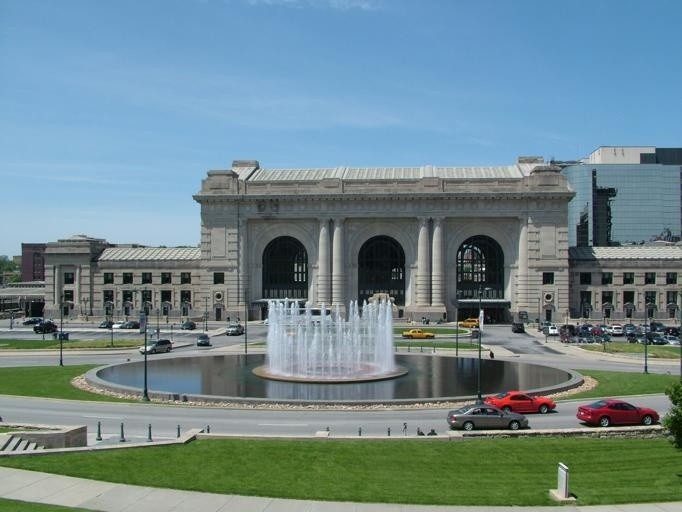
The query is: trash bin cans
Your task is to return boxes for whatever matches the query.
[52,332,69,340]
[472,330,480,339]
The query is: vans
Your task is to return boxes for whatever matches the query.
[140,338,173,354]
[512,322,524,333]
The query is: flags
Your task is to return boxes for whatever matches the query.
[478,309,485,333]
[139,310,147,334]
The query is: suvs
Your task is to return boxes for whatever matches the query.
[459,318,479,329]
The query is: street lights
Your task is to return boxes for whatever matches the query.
[477,285,493,406]
[604,315,608,351]
[644,292,653,374]
[456,289,467,358]
[536,297,541,332]
[140,286,153,402]
[203,296,211,331]
[59,292,72,368]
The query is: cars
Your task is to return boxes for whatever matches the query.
[402,329,436,340]
[197,333,210,347]
[22,318,42,325]
[182,321,196,330]
[576,399,660,427]
[100,320,140,330]
[447,405,530,431]
[226,324,245,335]
[34,320,57,335]
[543,324,681,347]
[483,392,557,413]
